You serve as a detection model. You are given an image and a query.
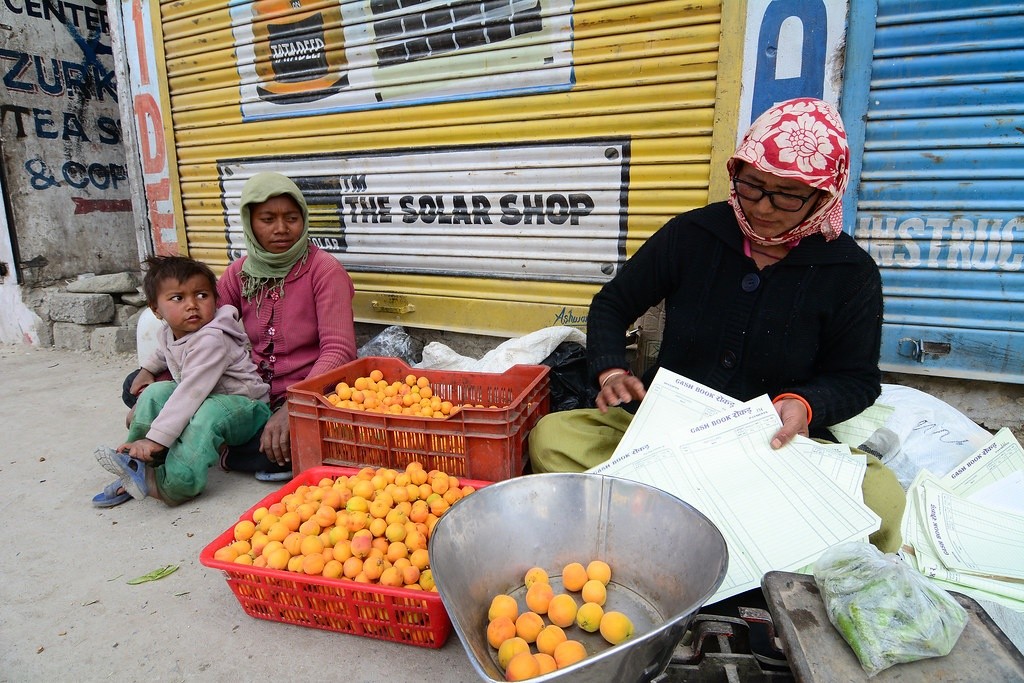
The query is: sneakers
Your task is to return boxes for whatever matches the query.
[255,465,293,481]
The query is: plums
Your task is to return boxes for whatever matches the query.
[214,368,638,682]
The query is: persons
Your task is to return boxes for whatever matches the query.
[122,171,358,481]
[91,254,274,509]
[531,97,907,557]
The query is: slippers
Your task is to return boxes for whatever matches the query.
[93,477,131,507]
[94,446,148,501]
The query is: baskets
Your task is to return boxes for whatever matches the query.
[199,466,494,651]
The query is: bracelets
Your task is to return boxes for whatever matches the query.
[602,372,629,387]
[774,394,813,426]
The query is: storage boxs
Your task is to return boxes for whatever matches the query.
[283,355,554,482]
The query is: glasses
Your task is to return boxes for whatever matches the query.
[734,169,819,213]
